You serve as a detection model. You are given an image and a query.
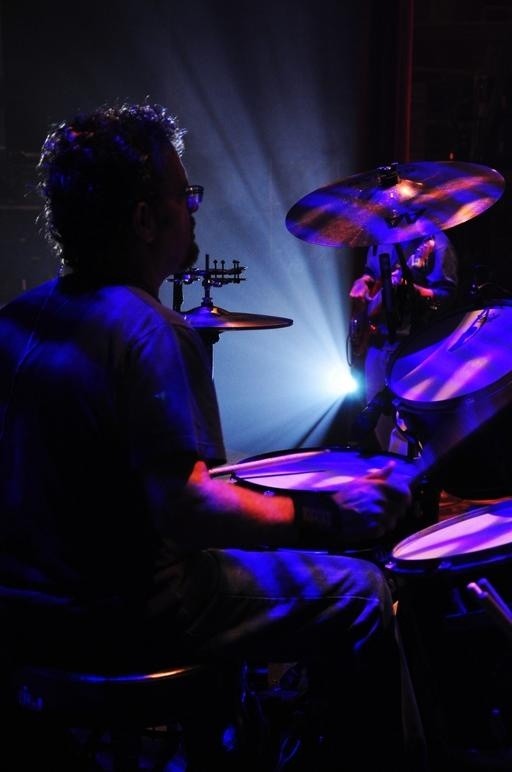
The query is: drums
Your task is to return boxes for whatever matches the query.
[230,445,440,555]
[383,300,511,451]
[391,499,511,619]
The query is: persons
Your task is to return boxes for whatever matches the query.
[0,104,425,770]
[350,229,457,401]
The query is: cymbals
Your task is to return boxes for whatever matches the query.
[284,160,506,246]
[181,305,292,331]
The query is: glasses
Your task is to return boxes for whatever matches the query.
[159,184,205,215]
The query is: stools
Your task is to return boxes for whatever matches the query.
[53,661,225,766]
